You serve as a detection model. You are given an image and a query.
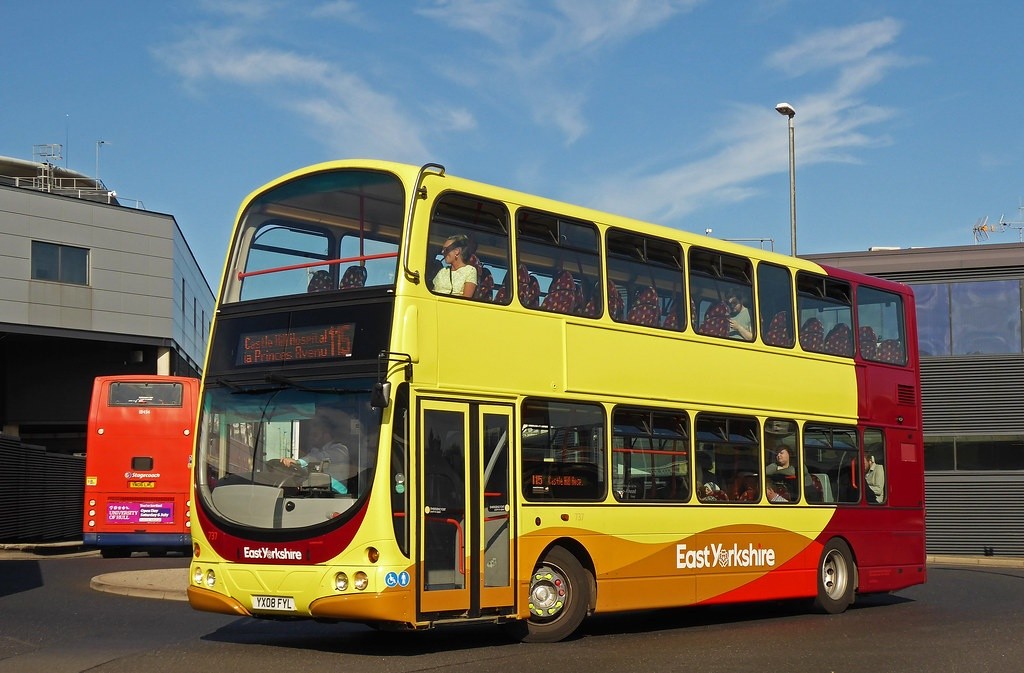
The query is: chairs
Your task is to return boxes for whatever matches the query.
[428,256,904,368]
[695,471,835,503]
[339,265,368,290]
[307,269,333,294]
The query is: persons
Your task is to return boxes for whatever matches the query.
[696,451,720,486]
[725,287,753,340]
[743,444,817,502]
[279,420,350,495]
[847,450,884,504]
[431,235,478,298]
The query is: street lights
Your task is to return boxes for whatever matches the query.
[774,102,797,260]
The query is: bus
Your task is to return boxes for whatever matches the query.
[185,159,926,643]
[83,374,265,558]
